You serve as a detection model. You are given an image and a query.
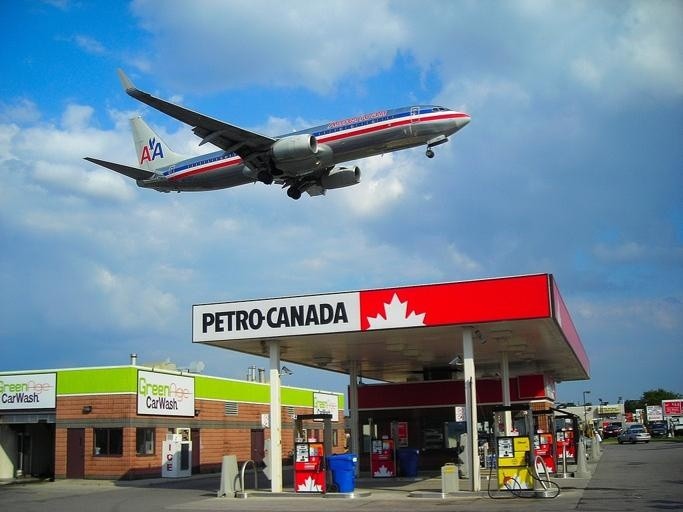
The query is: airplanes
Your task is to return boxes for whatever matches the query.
[82,66,473,207]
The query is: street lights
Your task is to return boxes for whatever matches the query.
[582,390,591,432]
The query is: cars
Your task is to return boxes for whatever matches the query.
[646,419,667,438]
[602,423,622,439]
[627,424,648,434]
[617,428,651,444]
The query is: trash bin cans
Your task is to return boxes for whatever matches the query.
[325,454,358,493]
[398,447,420,478]
